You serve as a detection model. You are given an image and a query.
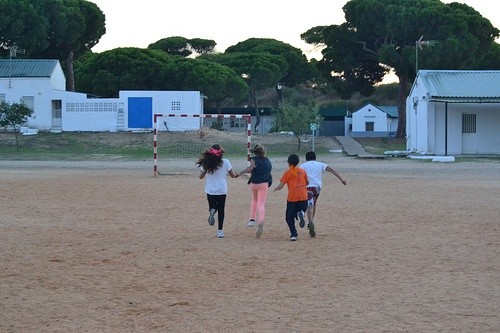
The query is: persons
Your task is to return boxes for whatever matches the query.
[300,151,347,238]
[194,143,238,238]
[236,142,273,239]
[272,153,310,241]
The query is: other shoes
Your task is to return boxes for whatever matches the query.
[248,219,255,226]
[308,222,316,237]
[256,222,263,236]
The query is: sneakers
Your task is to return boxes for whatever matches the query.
[208,208,215,226]
[290,234,298,240]
[299,211,305,228]
[218,230,224,238]
